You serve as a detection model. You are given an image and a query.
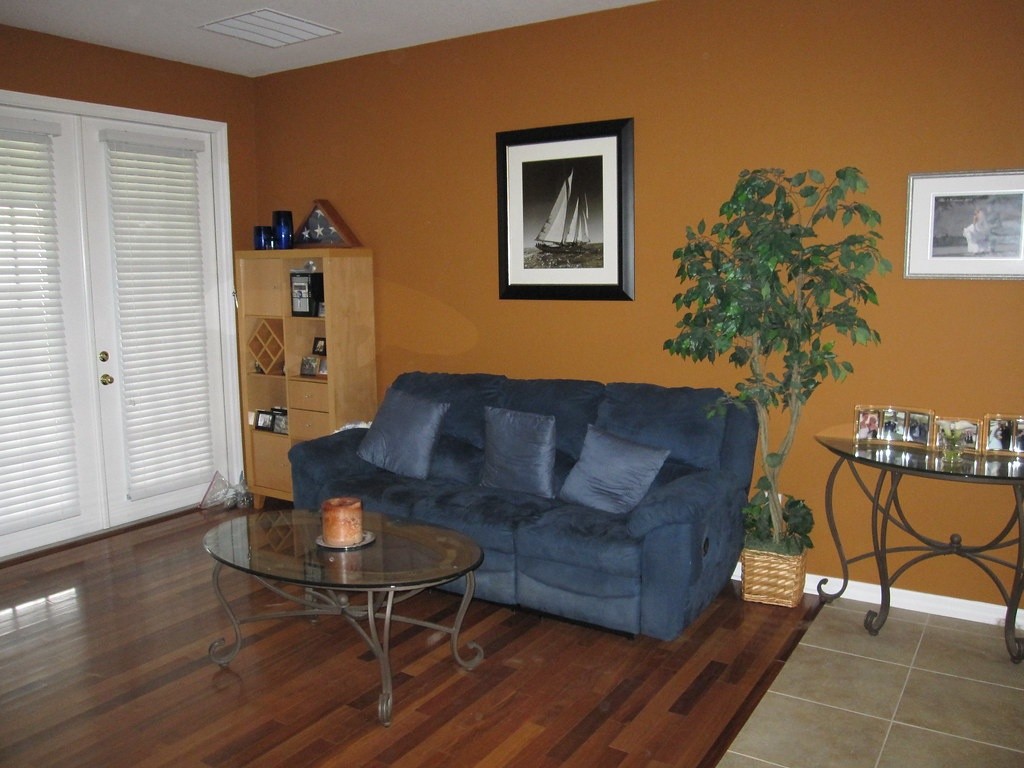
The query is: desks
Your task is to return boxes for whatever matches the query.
[205,509,486,725]
[814,424,1024,666]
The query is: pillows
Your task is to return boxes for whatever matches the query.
[559,423,671,512]
[481,405,559,500]
[356,385,454,483]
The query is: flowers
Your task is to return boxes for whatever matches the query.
[933,418,977,459]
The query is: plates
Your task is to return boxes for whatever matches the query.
[317,530,376,548]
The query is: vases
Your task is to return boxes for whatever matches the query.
[938,431,967,463]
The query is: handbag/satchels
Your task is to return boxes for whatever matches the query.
[199,470,254,510]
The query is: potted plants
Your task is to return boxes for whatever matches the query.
[664,164,897,610]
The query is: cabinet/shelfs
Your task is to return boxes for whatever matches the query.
[233,246,378,509]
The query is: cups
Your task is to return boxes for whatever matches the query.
[272,211,294,249]
[321,497,363,546]
[254,226,271,250]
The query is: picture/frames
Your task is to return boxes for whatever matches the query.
[902,172,1024,282]
[255,410,276,431]
[495,116,636,302]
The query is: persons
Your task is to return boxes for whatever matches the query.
[297,289,303,308]
[963,204,1002,255]
[856,412,1024,453]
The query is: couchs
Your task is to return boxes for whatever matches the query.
[288,370,761,644]
